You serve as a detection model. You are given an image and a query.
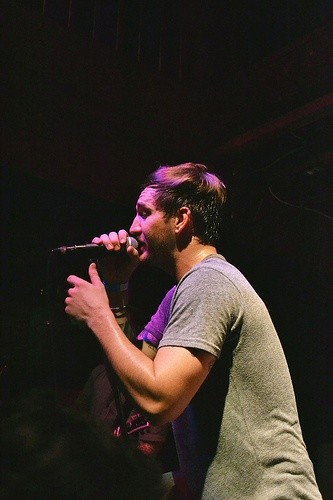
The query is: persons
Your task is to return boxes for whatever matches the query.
[76,261,174,463]
[64,161,325,500]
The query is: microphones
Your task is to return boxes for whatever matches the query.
[49,237,139,255]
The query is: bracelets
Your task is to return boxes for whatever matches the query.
[103,282,131,296]
